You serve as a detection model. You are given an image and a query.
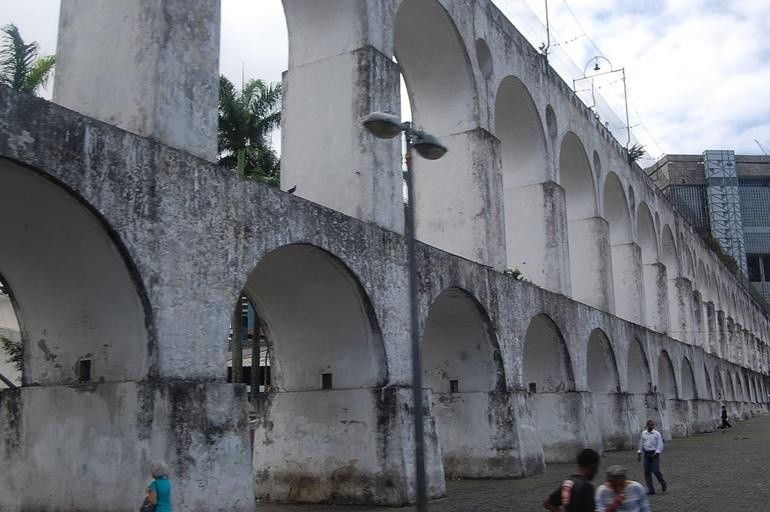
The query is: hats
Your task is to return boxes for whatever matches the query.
[605,465,628,481]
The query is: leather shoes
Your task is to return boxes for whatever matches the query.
[662,481,667,492]
[645,488,655,495]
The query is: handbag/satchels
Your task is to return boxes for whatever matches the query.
[139,481,158,512]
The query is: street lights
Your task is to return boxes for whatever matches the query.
[360,110,449,506]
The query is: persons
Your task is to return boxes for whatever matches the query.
[541,448,601,511]
[145,459,173,511]
[720,405,732,428]
[595,464,651,511]
[637,419,666,495]
